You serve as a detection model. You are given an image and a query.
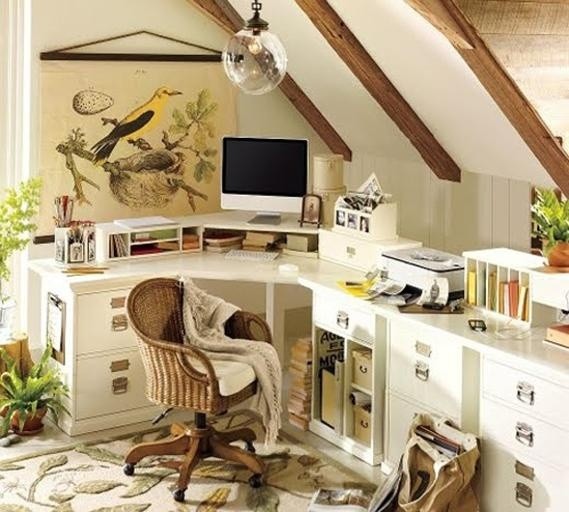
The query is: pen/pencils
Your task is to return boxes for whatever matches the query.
[62,266,109,277]
[53,196,73,228]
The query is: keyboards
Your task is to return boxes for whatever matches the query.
[226,251,276,261]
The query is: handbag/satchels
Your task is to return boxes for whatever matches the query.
[369,413,482,512]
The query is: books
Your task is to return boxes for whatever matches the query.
[204,232,318,257]
[308,453,403,512]
[110,230,199,257]
[288,337,311,432]
[545,325,569,348]
[468,271,529,321]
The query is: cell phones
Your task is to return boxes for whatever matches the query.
[469,317,486,332]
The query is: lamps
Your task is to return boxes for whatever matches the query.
[222,1,287,98]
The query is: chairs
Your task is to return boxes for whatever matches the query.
[119,276,283,502]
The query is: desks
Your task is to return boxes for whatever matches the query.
[29,258,298,436]
[299,259,569,512]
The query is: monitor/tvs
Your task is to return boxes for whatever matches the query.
[220,137,310,228]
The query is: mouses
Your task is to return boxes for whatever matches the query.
[279,263,300,272]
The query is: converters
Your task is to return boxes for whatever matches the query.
[559,309,567,315]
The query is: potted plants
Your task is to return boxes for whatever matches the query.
[528,184,569,268]
[0,348,66,441]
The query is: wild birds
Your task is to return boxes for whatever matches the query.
[89,84,185,170]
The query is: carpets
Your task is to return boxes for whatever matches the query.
[0,407,401,511]
